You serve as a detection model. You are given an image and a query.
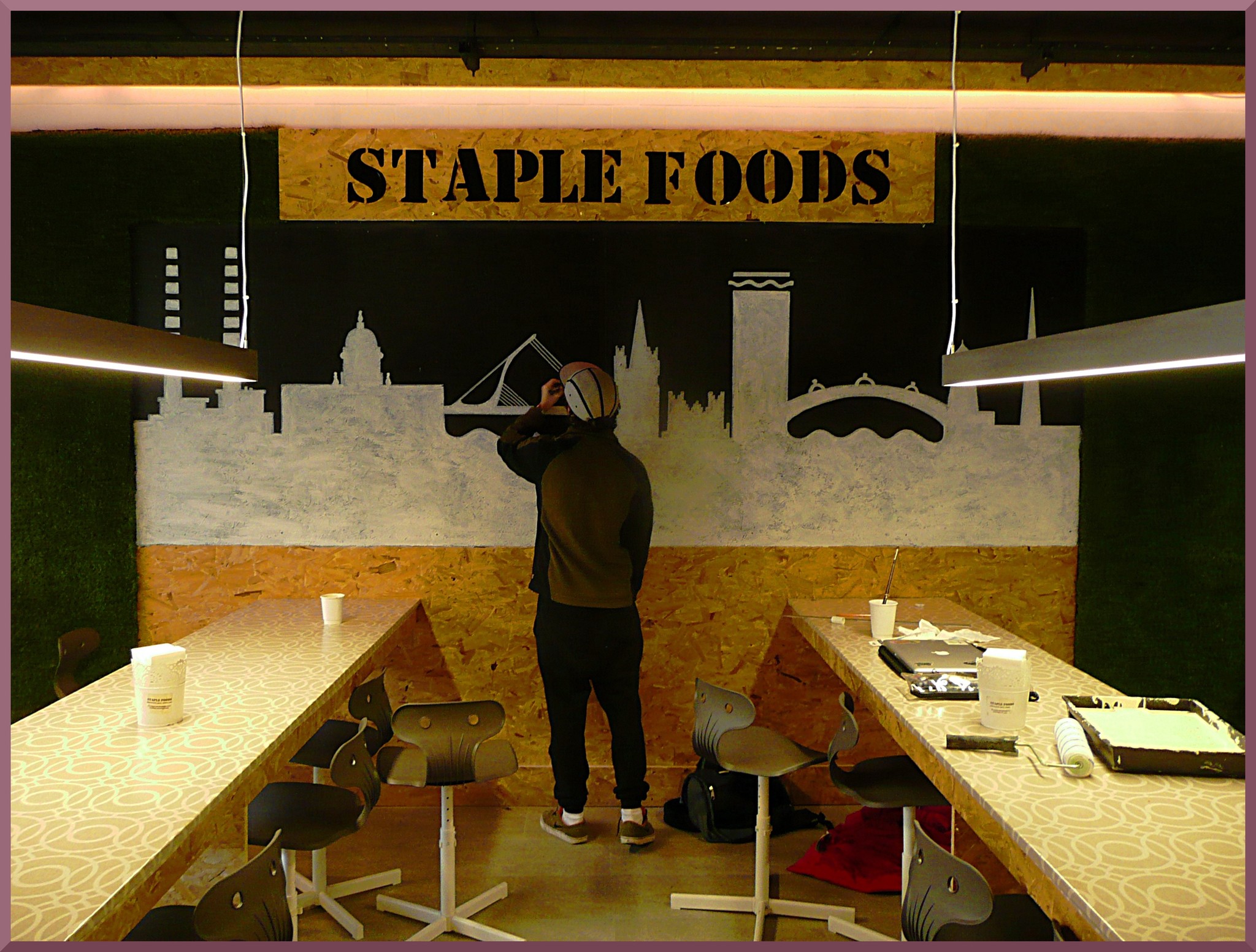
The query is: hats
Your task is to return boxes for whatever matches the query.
[559,362,620,423]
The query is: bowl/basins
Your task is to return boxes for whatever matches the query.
[134,682,184,727]
[978,686,1030,731]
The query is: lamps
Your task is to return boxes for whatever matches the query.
[11,11,259,384]
[942,11,1247,386]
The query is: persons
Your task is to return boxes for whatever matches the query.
[496,362,656,843]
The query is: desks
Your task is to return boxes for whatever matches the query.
[789,598,1245,941]
[11,596,422,941]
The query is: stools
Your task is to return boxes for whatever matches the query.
[902,819,1054,941]
[288,666,402,941]
[376,701,530,941]
[54,627,100,699]
[248,715,381,941]
[826,692,950,941]
[671,676,857,941]
[120,827,294,941]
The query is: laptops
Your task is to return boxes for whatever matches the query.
[882,640,987,674]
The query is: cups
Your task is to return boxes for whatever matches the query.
[869,599,898,639]
[320,593,345,624]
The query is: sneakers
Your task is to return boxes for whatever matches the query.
[616,806,656,843]
[539,805,588,844]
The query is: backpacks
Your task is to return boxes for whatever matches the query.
[682,767,817,844]
